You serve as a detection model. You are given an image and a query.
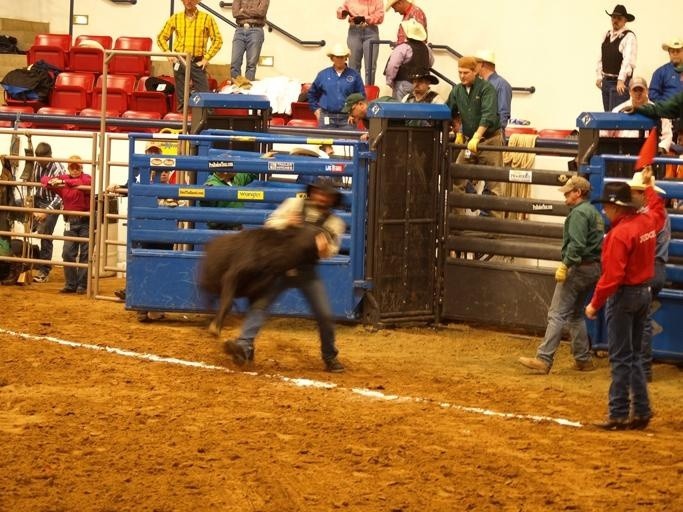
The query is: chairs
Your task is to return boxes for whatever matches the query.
[0,29,194,134]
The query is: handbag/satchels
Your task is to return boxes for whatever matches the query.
[0,65,53,104]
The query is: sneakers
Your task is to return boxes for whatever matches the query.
[34,273,49,283]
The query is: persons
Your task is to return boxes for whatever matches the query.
[648,42,683,150]
[199,152,279,231]
[231,0,269,86]
[386,0,428,48]
[402,72,445,126]
[106,145,179,318]
[307,51,366,129]
[337,0,385,85]
[289,145,335,185]
[42,156,91,292]
[14,145,67,282]
[344,94,400,140]
[225,173,347,376]
[629,172,672,383]
[445,50,509,237]
[519,176,604,373]
[0,154,14,283]
[618,93,683,291]
[586,167,665,430]
[612,78,672,178]
[160,0,223,114]
[596,6,639,134]
[387,19,435,101]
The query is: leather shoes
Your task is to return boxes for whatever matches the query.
[77,286,85,294]
[592,417,630,430]
[567,361,593,371]
[518,356,550,375]
[224,340,246,367]
[327,357,345,373]
[628,416,649,431]
[60,288,77,292]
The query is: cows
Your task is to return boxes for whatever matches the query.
[195,211,338,338]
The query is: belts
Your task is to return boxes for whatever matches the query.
[240,24,259,28]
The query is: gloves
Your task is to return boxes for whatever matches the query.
[555,267,566,282]
[467,133,481,153]
[353,16,366,25]
[342,10,348,17]
[455,133,464,144]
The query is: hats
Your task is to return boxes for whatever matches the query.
[629,78,646,92]
[290,145,329,158]
[306,179,343,207]
[69,155,82,168]
[35,143,51,156]
[327,42,351,58]
[384,0,496,84]
[145,142,163,152]
[340,93,365,113]
[605,4,634,22]
[557,172,668,209]
[662,36,683,52]
[145,78,174,94]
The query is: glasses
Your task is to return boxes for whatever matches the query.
[348,107,355,115]
